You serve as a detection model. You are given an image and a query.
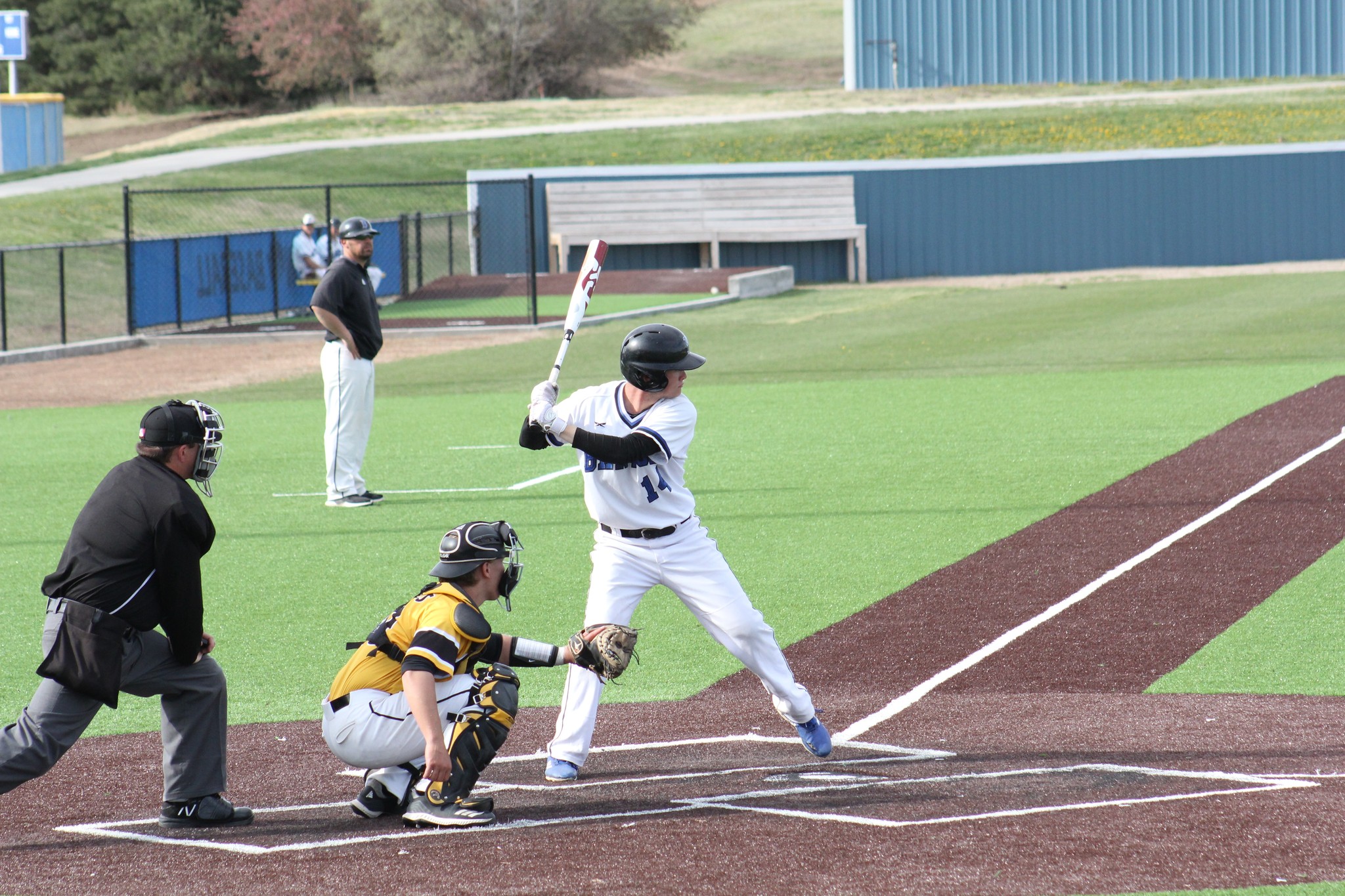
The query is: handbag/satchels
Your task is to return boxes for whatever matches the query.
[35,600,129,710]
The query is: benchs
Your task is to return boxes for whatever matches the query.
[546,174,868,285]
[293,278,321,288]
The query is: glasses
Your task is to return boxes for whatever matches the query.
[348,234,374,239]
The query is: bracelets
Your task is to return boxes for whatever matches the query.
[554,647,564,666]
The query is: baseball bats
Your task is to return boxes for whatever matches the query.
[530,236,609,437]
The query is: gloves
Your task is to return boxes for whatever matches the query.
[527,380,568,438]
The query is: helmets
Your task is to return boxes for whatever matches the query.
[428,521,507,579]
[339,216,379,239]
[620,323,707,393]
[330,218,340,226]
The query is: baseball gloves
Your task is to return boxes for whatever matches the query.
[566,623,645,687]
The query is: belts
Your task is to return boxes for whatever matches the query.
[600,516,690,539]
[330,693,350,713]
[47,598,133,641]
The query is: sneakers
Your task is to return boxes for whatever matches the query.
[776,708,832,757]
[158,794,255,830]
[401,787,496,828]
[325,491,384,507]
[545,756,578,782]
[351,778,398,818]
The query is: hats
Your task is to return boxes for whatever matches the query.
[302,213,315,225]
[139,400,223,447]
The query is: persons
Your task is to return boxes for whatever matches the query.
[309,216,384,508]
[291,213,384,310]
[0,399,256,828]
[322,520,607,827]
[519,324,832,782]
